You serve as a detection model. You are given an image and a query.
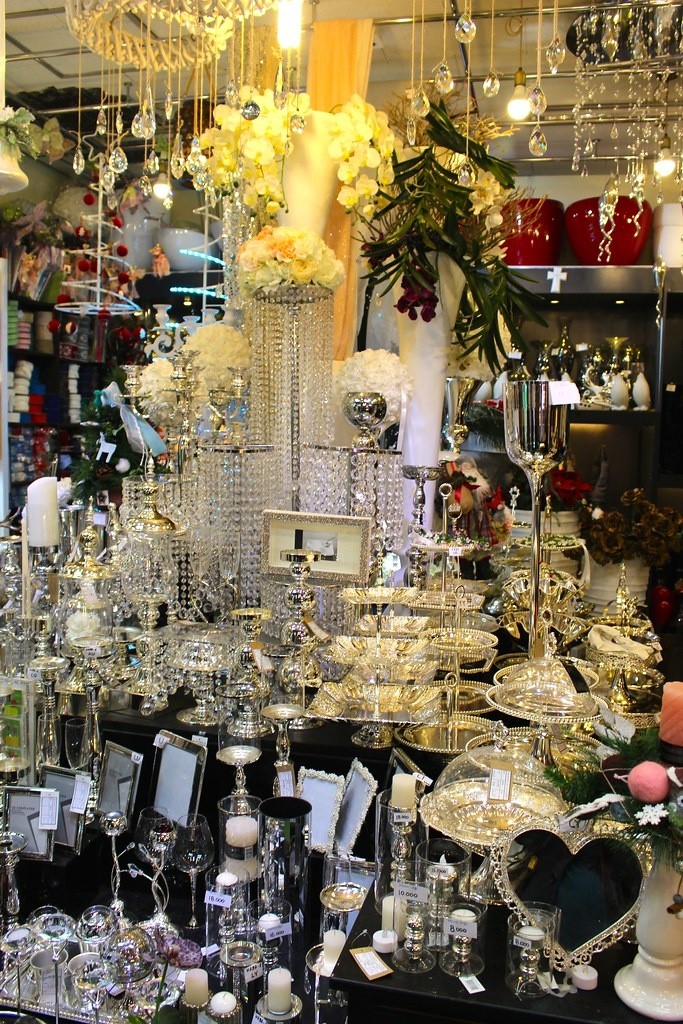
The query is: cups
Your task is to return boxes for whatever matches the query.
[68,951,101,1013]
[205,865,249,977]
[245,897,293,990]
[506,913,555,997]
[30,946,68,1005]
[372,787,428,916]
[415,837,471,952]
[437,892,488,977]
[507,508,578,579]
[579,548,649,615]
[652,203,682,267]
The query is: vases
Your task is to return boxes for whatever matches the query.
[277,107,342,239]
[613,839,683,1022]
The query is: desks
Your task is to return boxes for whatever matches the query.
[328,881,683,1023]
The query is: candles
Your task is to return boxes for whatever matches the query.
[660,681,683,746]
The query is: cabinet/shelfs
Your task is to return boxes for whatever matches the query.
[3,290,130,425]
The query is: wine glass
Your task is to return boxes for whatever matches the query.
[0,347,571,1024]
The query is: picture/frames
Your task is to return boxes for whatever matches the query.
[261,509,369,582]
[4,729,431,935]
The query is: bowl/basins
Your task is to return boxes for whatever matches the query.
[54,185,224,271]
[564,194,651,265]
[502,194,564,264]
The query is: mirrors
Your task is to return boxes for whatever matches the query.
[486,821,653,975]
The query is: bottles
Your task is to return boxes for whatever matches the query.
[530,316,650,410]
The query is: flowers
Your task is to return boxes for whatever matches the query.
[544,700,682,916]
[197,85,551,385]
